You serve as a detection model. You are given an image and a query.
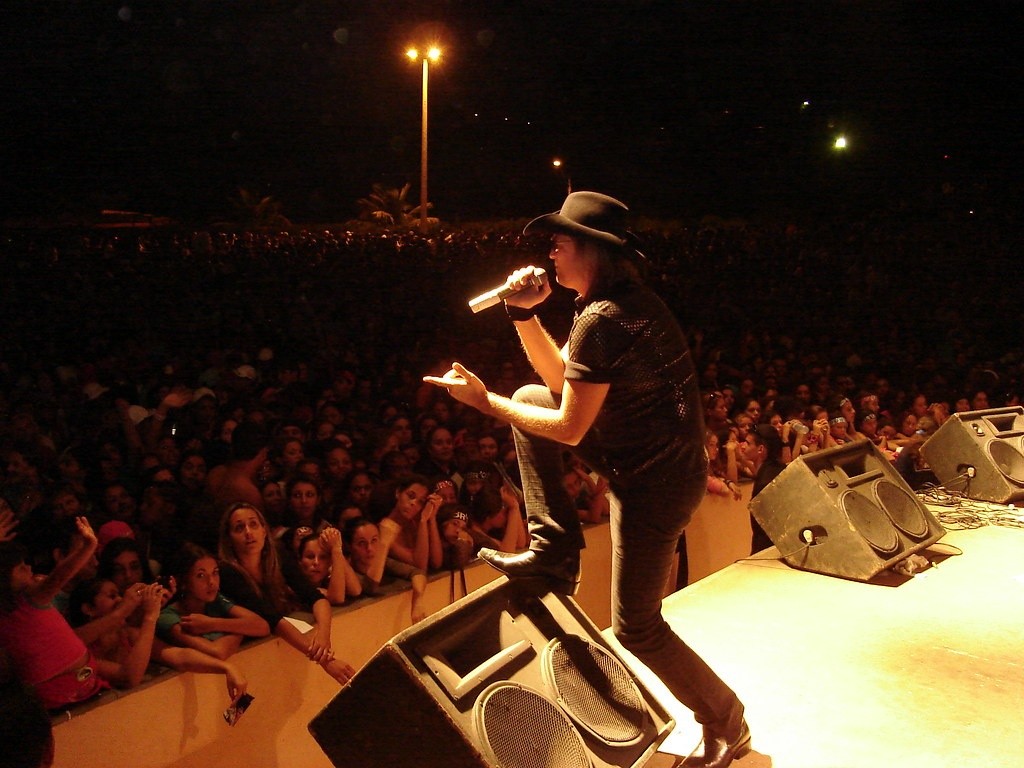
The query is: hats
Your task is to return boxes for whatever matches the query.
[257,346,273,362]
[522,190,655,261]
[233,363,257,381]
[127,404,157,426]
[79,380,110,404]
[191,386,216,403]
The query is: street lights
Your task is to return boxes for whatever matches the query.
[406,46,442,236]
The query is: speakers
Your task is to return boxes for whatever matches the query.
[307,571,676,768]
[747,437,947,583]
[919,405,1024,505]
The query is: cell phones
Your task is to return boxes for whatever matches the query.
[155,576,171,592]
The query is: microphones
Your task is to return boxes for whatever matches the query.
[469,269,548,314]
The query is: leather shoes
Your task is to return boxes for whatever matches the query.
[476,546,582,596]
[683,717,752,768]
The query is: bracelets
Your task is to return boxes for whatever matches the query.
[505,305,535,321]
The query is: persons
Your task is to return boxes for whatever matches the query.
[0,205,1023,767]
[423,192,751,768]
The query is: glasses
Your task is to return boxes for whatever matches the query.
[551,241,577,253]
